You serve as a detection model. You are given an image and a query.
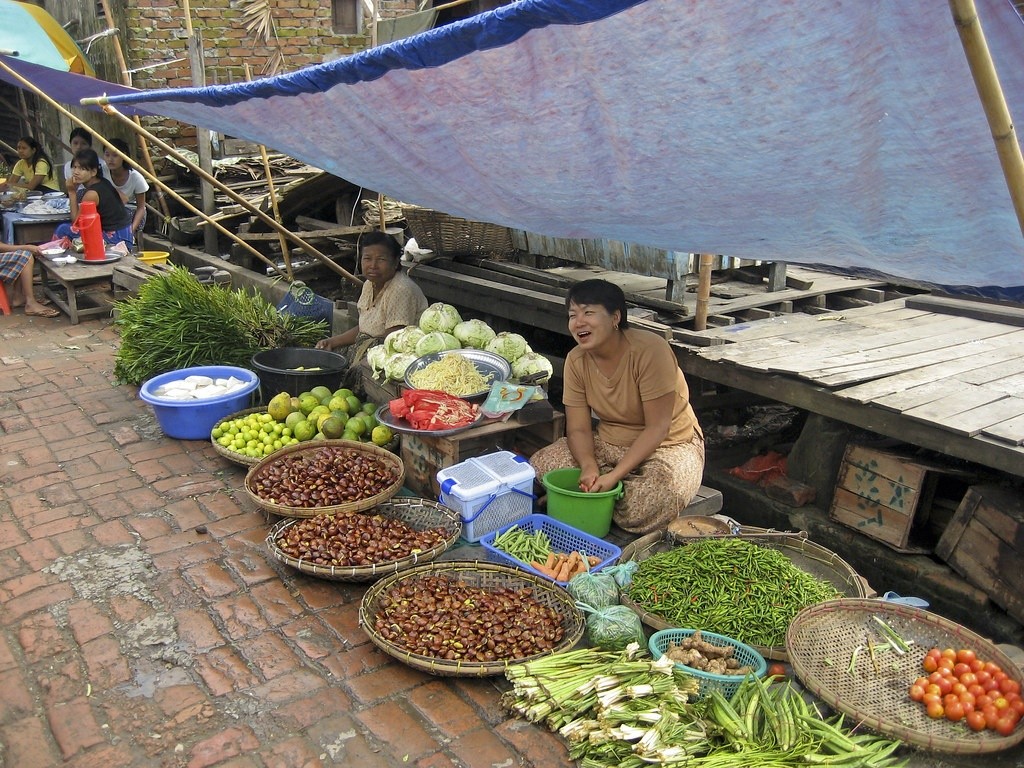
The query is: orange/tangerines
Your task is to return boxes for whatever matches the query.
[268,385,395,447]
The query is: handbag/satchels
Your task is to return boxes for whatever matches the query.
[276,280,333,330]
[41,192,67,201]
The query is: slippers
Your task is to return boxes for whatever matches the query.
[21,297,51,306]
[26,306,61,318]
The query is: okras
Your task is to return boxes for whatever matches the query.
[709,667,909,768]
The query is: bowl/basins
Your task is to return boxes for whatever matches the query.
[135,251,170,266]
[138,366,261,441]
[250,348,348,406]
[40,249,66,261]
[52,258,67,268]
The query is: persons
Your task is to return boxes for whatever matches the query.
[101,139,150,239]
[314,231,428,400]
[0,135,60,195]
[0,242,60,318]
[64,128,108,200]
[51,148,133,251]
[528,279,704,534]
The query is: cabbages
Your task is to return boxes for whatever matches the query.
[367,303,553,384]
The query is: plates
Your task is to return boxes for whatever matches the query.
[404,348,510,401]
[375,404,484,436]
[0,191,67,200]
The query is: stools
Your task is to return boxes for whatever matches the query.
[0,280,12,315]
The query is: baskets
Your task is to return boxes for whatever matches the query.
[480,514,622,586]
[648,628,767,702]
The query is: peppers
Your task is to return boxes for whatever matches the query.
[565,535,848,651]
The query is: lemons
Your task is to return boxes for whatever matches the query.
[210,412,300,458]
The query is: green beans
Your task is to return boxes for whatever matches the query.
[491,524,554,566]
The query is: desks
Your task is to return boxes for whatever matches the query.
[35,247,146,325]
[0,211,71,246]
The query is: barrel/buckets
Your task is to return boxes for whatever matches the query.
[539,468,625,539]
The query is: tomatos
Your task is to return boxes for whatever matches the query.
[910,648,1024,735]
[768,664,786,681]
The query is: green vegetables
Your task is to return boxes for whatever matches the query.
[501,647,719,768]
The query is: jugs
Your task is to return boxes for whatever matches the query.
[72,202,105,260]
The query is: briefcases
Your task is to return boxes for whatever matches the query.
[435,449,535,542]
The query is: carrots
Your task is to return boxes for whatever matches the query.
[531,550,602,581]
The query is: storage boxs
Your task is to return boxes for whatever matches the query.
[364,360,1023,625]
